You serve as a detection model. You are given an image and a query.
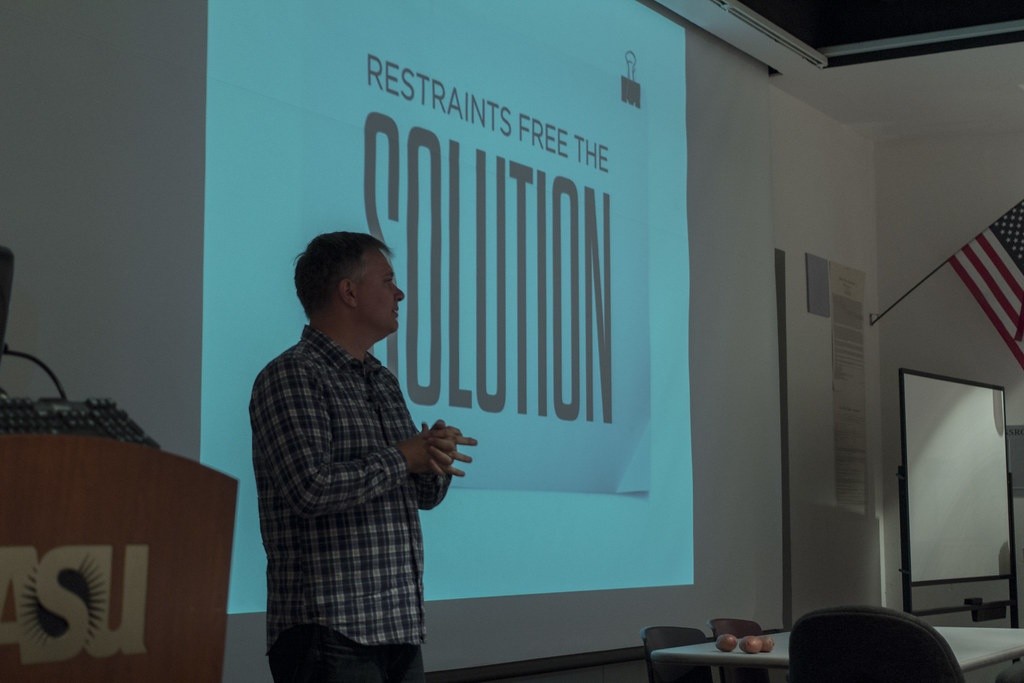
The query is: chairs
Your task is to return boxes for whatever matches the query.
[707,617,769,683]
[785,604,966,683]
[640,625,713,683]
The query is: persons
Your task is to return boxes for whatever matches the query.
[250,233,477,683]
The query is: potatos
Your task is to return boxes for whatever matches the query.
[716,634,776,653]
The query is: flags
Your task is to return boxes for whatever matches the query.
[949,200,1024,370]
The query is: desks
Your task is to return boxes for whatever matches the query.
[650,626,1024,683]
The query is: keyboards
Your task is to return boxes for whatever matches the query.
[0,394,161,451]
